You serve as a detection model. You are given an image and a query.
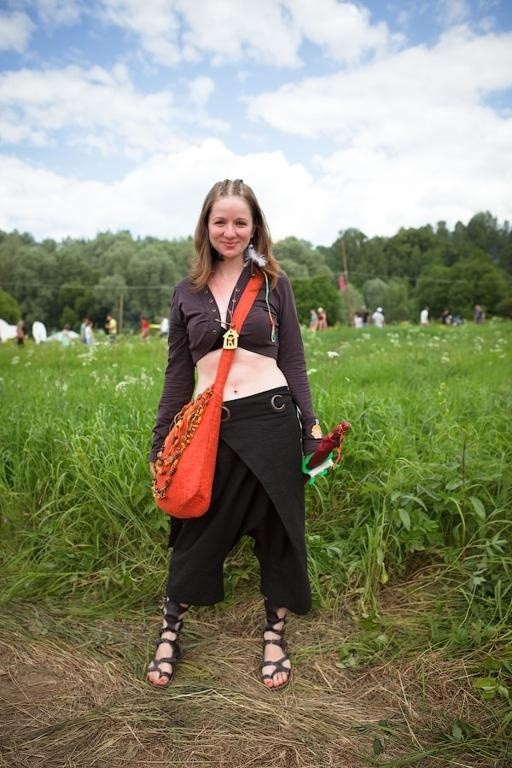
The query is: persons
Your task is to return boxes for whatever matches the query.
[305,302,485,333]
[144,177,333,692]
[15,314,172,350]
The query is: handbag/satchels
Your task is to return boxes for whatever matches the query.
[151,388,224,518]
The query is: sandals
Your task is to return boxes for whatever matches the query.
[150,599,188,687]
[261,610,289,693]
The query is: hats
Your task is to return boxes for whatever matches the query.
[377,307,383,312]
[318,307,324,313]
